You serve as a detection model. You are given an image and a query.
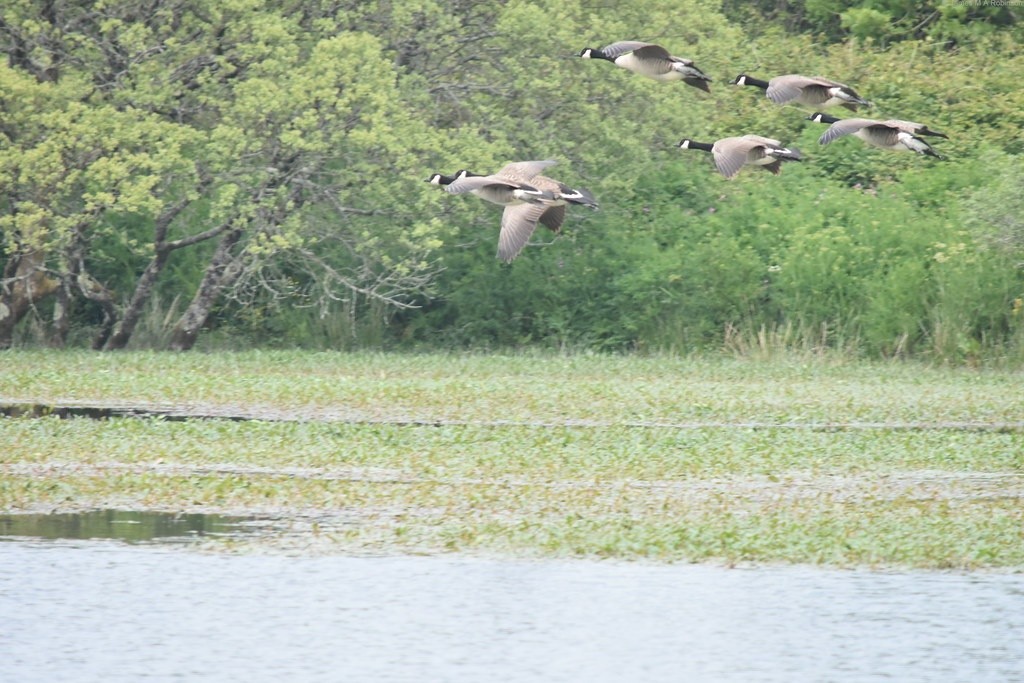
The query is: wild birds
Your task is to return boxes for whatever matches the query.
[574,38,947,165]
[424,162,599,265]
